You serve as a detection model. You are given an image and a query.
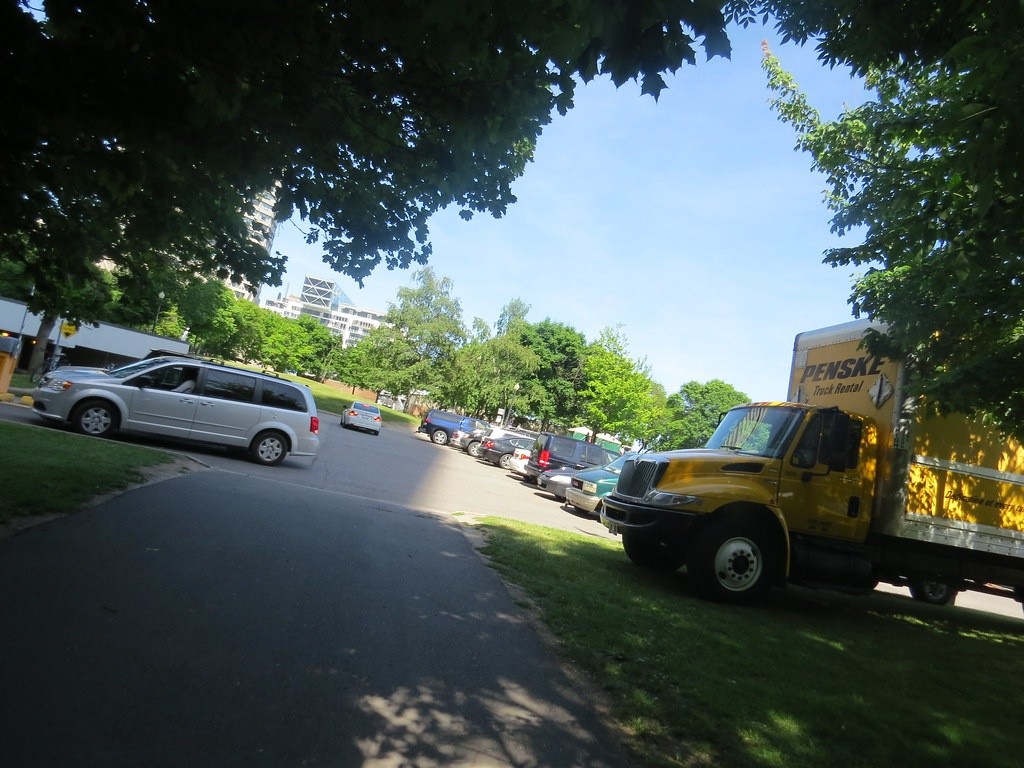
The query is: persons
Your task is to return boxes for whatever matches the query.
[171,367,198,394]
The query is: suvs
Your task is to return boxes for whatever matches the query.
[414,408,490,445]
[30,355,319,466]
[460,429,484,457]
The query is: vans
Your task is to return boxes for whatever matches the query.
[524,431,620,481]
[565,453,638,513]
[480,428,536,449]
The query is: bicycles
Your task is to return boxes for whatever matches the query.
[31,347,65,383]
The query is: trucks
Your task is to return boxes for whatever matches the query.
[599,318,1024,609]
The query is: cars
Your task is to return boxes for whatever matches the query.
[537,465,579,499]
[510,445,536,481]
[476,436,535,470]
[341,401,381,436]
